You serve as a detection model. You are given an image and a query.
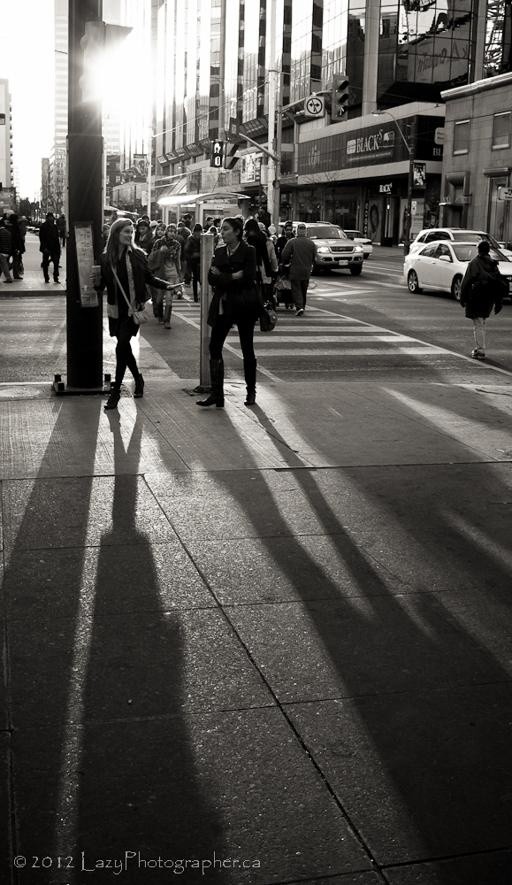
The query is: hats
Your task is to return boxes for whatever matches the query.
[45,211,55,219]
[478,242,490,250]
[297,224,307,235]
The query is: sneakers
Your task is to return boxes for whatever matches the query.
[4,280,12,283]
[194,297,198,302]
[159,317,165,325]
[295,308,304,316]
[54,279,60,284]
[14,276,23,279]
[45,278,49,283]
[165,320,171,328]
[472,349,486,359]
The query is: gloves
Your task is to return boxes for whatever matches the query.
[494,304,504,315]
[459,298,466,308]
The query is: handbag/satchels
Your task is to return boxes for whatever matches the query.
[133,311,149,325]
[260,309,277,332]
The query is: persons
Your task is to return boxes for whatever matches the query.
[0,208,316,330]
[457,240,504,360]
[369,205,382,244]
[195,216,264,410]
[91,219,188,411]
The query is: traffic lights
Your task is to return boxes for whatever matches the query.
[332,73,350,121]
[210,140,224,168]
[223,141,240,169]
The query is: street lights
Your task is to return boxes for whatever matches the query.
[370,109,414,260]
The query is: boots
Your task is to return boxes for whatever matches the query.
[134,374,144,398]
[196,359,224,407]
[104,388,120,410]
[243,358,256,405]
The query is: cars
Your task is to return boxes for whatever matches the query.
[269,217,373,276]
[402,226,512,305]
